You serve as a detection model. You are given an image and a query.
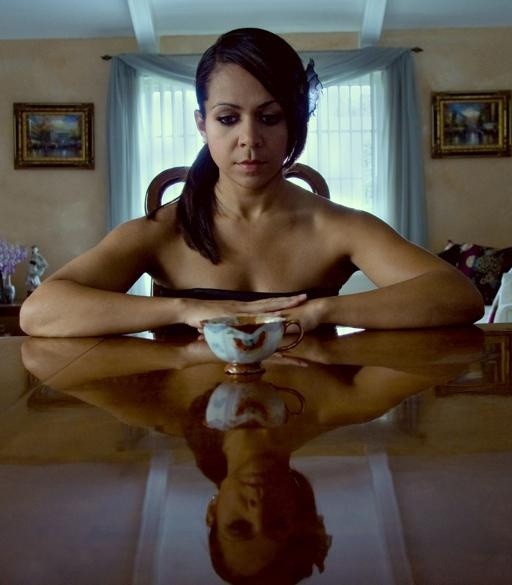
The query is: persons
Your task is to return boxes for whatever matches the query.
[18,27,486,338]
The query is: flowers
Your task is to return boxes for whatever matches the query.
[0,241,27,275]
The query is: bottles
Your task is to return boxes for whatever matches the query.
[201,379,307,430]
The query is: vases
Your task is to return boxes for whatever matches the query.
[1,270,15,303]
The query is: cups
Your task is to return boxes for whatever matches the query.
[200,315,304,376]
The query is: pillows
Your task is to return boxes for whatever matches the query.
[437,240,510,307]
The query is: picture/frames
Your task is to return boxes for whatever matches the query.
[13,101,95,168]
[430,89,512,158]
